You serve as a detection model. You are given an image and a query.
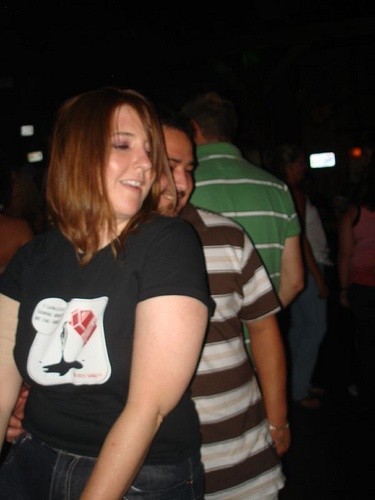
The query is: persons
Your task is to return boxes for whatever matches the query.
[0,86,218,500]
[181,93,305,371]
[264,128,375,410]
[152,114,292,500]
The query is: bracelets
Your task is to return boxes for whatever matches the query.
[338,288,349,292]
[268,422,291,431]
[310,265,320,273]
